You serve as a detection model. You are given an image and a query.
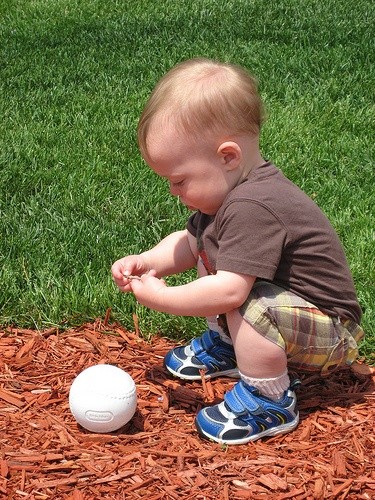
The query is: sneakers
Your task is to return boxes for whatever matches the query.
[163,329,241,380]
[194,378,302,445]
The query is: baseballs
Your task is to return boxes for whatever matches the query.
[68,365,137,433]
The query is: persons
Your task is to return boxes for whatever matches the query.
[111,60,365,444]
